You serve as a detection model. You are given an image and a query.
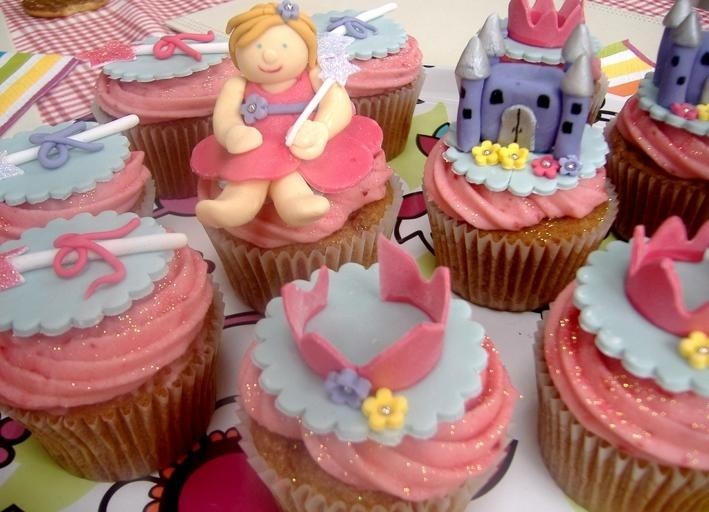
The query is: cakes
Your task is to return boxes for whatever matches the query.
[0,0,709,512]
